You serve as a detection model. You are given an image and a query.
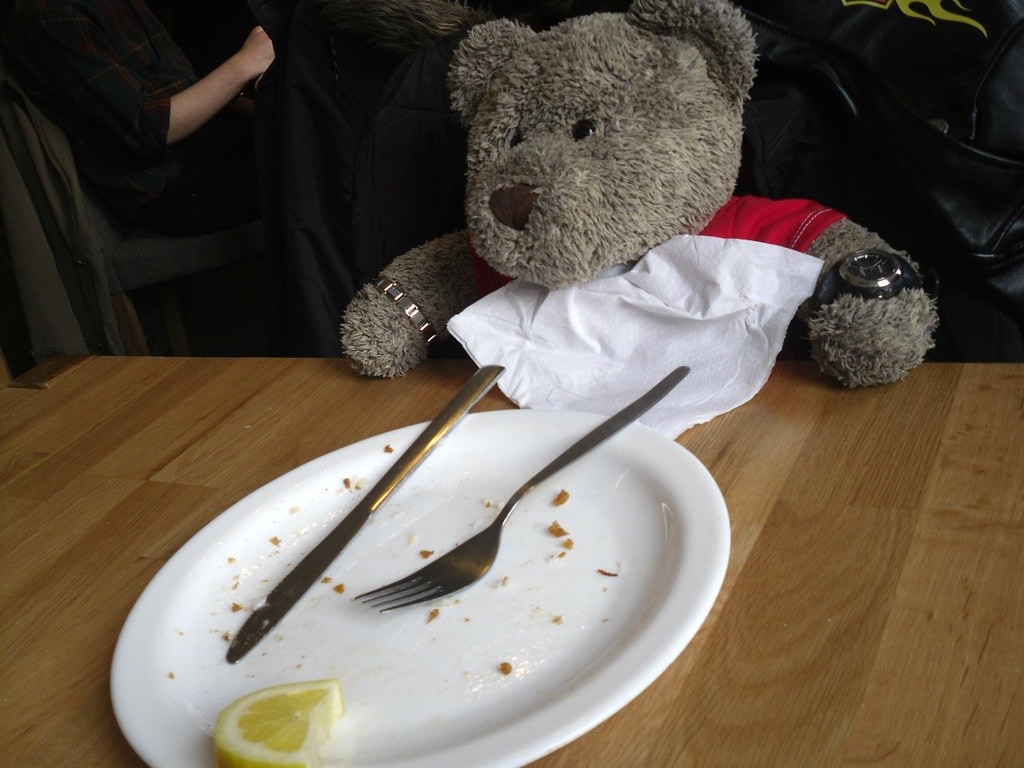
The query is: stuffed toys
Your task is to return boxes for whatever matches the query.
[323,0,940,387]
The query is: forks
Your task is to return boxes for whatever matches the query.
[352,366,691,612]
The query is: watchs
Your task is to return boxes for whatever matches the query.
[785,250,927,361]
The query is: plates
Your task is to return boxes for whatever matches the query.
[110,409,727,768]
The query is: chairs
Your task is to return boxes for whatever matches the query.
[79,191,265,356]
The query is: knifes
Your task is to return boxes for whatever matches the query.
[226,366,508,663]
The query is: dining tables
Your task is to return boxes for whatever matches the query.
[0,354,1023,768]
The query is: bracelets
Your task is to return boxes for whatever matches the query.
[372,275,441,349]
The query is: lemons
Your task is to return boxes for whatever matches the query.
[208,678,344,768]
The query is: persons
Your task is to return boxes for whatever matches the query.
[2,0,276,239]
